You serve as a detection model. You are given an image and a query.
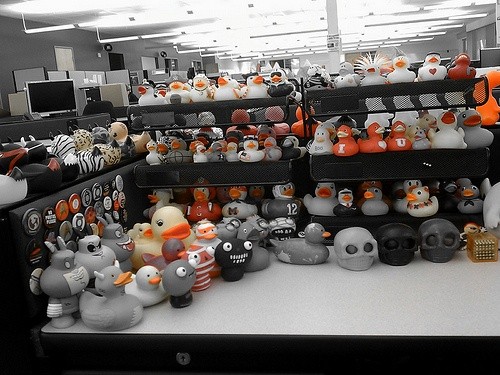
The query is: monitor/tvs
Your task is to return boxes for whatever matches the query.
[26,79,77,121]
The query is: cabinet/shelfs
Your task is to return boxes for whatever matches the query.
[127,78,492,247]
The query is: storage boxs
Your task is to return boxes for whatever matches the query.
[466,232,498,263]
